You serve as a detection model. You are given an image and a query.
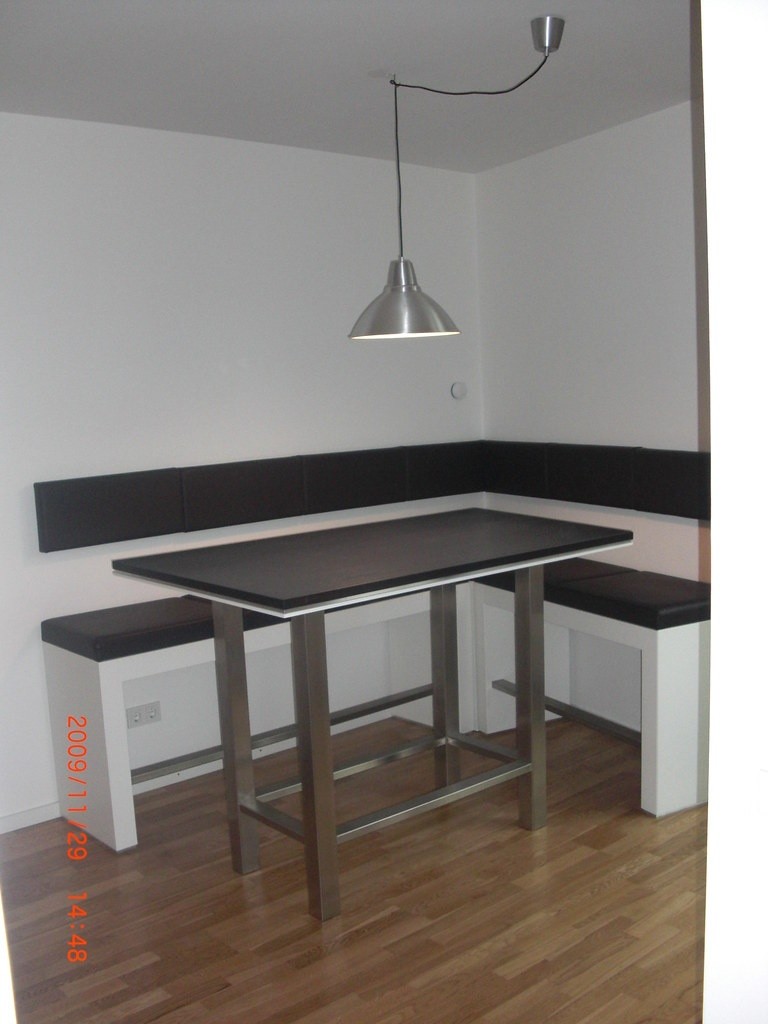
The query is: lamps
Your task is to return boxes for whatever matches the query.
[346,16,563,341]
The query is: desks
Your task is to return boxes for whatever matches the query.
[111,507,637,922]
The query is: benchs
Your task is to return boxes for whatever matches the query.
[472,557,710,817]
[41,577,476,853]
